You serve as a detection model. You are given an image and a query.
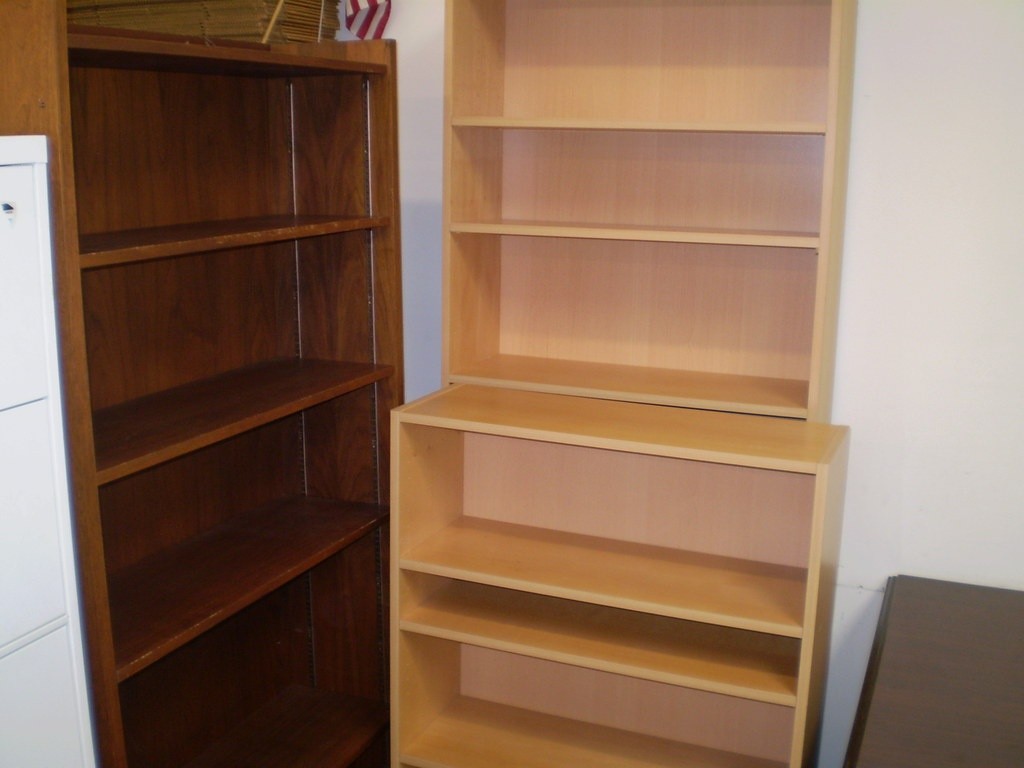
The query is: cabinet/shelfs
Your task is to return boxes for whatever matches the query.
[1,1,403,768]
[441,0,857,423]
[390,384,850,768]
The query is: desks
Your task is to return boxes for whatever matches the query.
[843,575,1024,768]
